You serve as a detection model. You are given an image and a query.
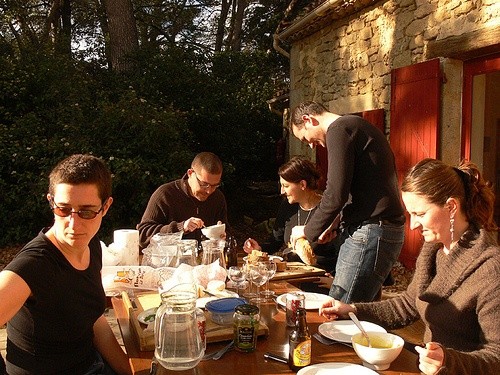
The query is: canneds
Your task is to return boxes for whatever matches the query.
[233,304,260,352]
[196,309,206,351]
[286,290,306,327]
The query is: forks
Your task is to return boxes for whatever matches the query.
[313,333,352,348]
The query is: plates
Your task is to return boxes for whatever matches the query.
[243,255,283,264]
[277,291,335,309]
[319,319,387,344]
[195,289,239,308]
[138,307,159,326]
[296,362,387,375]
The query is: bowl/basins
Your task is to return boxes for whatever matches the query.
[201,223,226,240]
[351,331,406,371]
[141,246,198,265]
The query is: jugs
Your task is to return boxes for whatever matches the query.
[154,291,204,371]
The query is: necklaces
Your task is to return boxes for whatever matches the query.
[298,210,311,225]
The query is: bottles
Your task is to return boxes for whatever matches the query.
[226,236,238,269]
[289,306,312,373]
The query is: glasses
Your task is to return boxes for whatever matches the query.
[51,198,108,219]
[195,172,221,188]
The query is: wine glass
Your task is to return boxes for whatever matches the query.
[228,260,277,305]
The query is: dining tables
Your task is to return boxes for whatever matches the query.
[110,262,419,375]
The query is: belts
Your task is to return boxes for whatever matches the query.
[358,214,406,226]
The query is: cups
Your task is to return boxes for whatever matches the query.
[173,238,227,270]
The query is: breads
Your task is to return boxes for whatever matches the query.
[295,238,317,266]
[196,280,225,298]
[247,250,287,271]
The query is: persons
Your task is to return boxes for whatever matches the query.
[291,101,406,303]
[242,155,340,275]
[319,158,500,375]
[0,154,134,375]
[137,152,229,253]
